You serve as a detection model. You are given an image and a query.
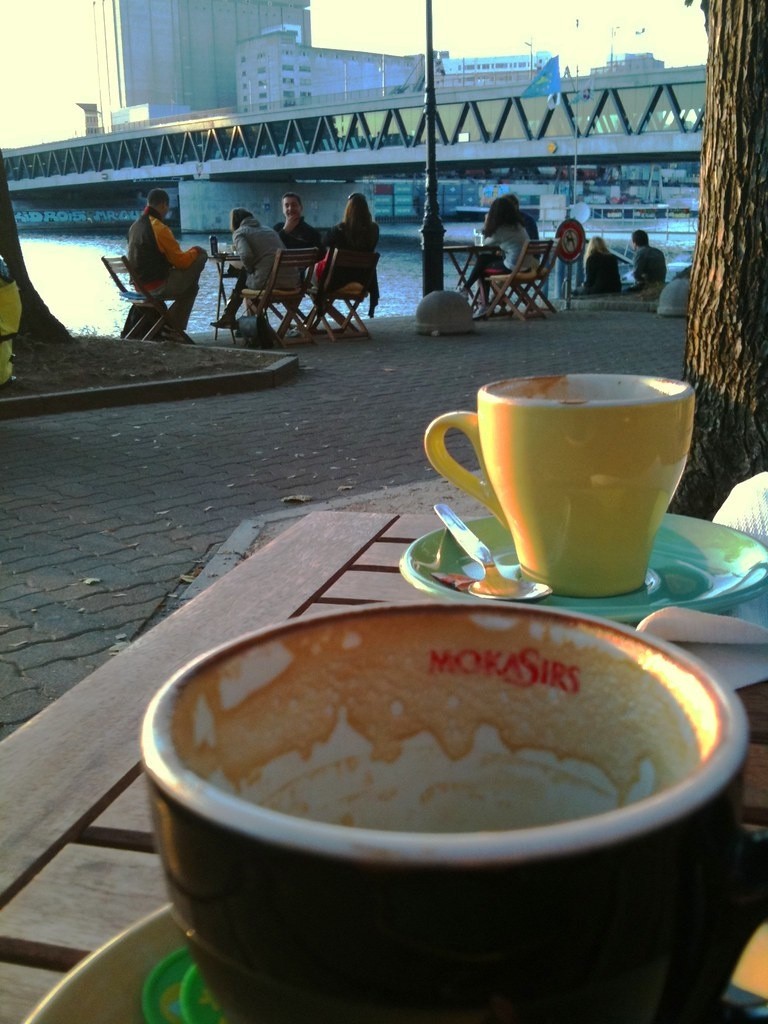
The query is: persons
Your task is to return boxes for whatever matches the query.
[273,192,323,253]
[304,192,380,293]
[210,207,303,330]
[622,229,667,293]
[447,194,542,319]
[570,236,622,296]
[125,188,208,346]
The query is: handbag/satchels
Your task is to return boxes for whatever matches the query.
[236,301,274,349]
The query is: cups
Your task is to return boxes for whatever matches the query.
[137,599,747,1023]
[423,373,696,599]
[217,243,227,254]
[474,228,484,246]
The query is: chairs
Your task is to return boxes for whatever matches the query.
[303,248,382,343]
[100,255,195,346]
[239,247,320,351]
[483,234,561,320]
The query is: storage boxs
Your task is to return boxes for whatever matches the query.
[365,180,542,218]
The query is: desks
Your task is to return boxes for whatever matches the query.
[442,240,556,313]
[208,253,244,342]
[0,510,768,1023]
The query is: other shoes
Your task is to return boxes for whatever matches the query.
[161,324,195,346]
[493,305,507,314]
[471,302,491,319]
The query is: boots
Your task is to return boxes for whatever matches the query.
[210,289,245,328]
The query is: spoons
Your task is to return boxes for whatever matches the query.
[433,500,554,602]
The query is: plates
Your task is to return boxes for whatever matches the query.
[17,893,768,1024]
[400,510,768,621]
